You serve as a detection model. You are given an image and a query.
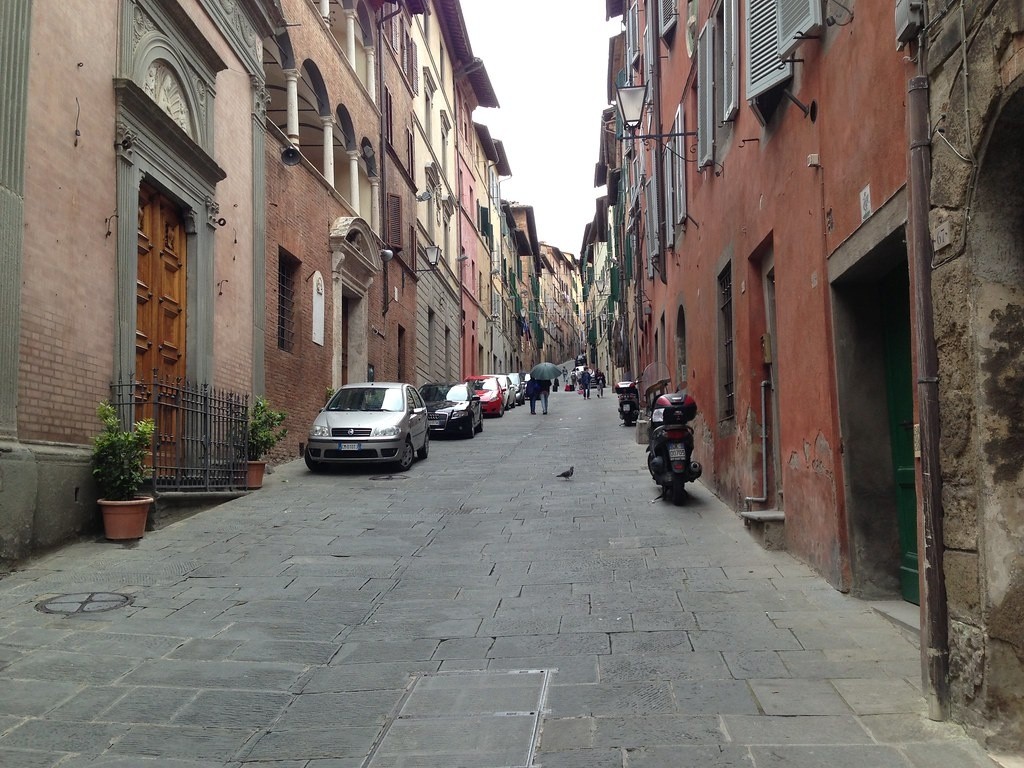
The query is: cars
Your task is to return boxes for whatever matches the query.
[518,370,531,397]
[573,354,596,387]
[495,372,525,406]
[304,382,430,474]
[417,380,484,439]
[475,374,518,411]
[463,375,507,417]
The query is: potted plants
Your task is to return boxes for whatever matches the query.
[225,393,291,489]
[88,398,161,542]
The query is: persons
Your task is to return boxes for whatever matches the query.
[526,377,540,414]
[562,366,568,382]
[554,377,559,392]
[537,379,551,415]
[571,368,582,386]
[582,367,592,399]
[595,368,606,398]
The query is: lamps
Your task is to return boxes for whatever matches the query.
[282,143,301,166]
[379,246,394,263]
[417,191,433,202]
[363,144,377,159]
[402,240,444,289]
[490,268,499,275]
[456,254,469,263]
[613,69,697,162]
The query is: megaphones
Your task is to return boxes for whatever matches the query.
[380,249,393,262]
[280,146,301,166]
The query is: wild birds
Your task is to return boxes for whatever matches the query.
[556,466,574,482]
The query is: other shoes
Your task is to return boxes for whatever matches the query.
[584,397,587,400]
[531,412,536,414]
[543,412,547,414]
[587,397,591,399]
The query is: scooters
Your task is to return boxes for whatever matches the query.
[641,362,703,507]
[614,370,648,427]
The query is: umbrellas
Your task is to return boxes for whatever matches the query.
[530,361,562,380]
